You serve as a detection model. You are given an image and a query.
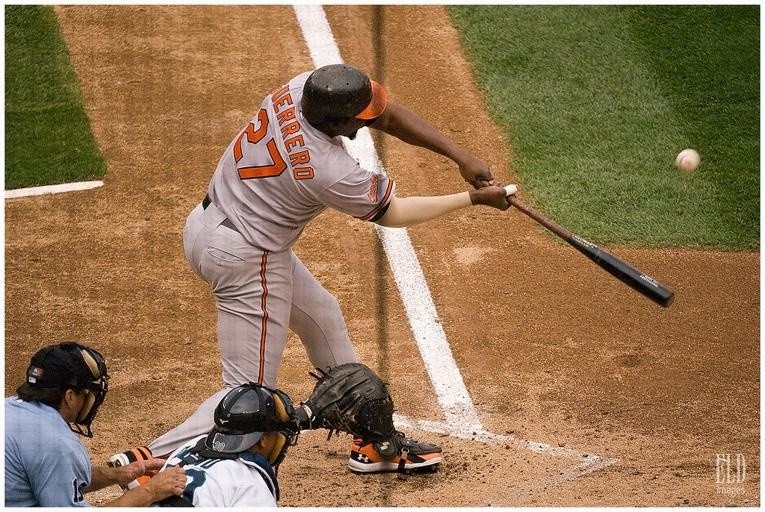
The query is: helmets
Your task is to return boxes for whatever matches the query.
[204,382,291,453]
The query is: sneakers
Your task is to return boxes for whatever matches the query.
[349,429,443,473]
[105,445,157,496]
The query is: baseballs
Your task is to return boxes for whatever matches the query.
[675,148,701,172]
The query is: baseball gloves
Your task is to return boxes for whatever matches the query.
[300,364,395,441]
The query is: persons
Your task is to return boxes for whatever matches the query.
[5,342,397,507]
[106,64,520,493]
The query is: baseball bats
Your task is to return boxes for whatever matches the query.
[481,181,675,308]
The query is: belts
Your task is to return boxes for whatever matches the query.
[199,191,243,236]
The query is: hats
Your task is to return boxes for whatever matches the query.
[302,60,386,125]
[15,340,110,403]
